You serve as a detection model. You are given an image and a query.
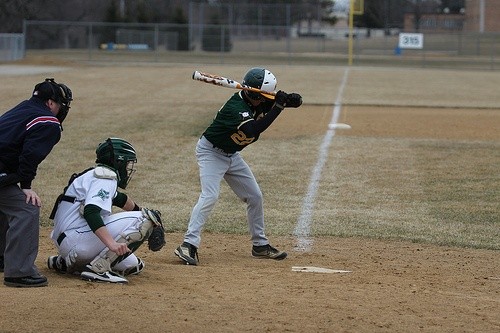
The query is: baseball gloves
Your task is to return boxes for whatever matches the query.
[149,212,166,253]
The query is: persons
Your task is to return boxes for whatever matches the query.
[174,67,303,265]
[105,2,231,52]
[47,137,164,283]
[0,78,72,287]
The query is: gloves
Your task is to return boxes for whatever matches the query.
[287,94,301,106]
[275,90,287,106]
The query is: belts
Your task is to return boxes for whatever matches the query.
[57,232,67,246]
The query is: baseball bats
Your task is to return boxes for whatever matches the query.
[192,69,275,95]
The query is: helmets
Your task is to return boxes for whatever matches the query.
[95,137,138,189]
[244,68,277,99]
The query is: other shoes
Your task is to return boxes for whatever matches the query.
[4,276,48,287]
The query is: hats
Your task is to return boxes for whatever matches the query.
[28,82,65,105]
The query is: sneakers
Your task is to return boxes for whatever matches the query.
[81,263,128,283]
[251,243,288,260]
[174,242,199,265]
[48,255,62,270]
